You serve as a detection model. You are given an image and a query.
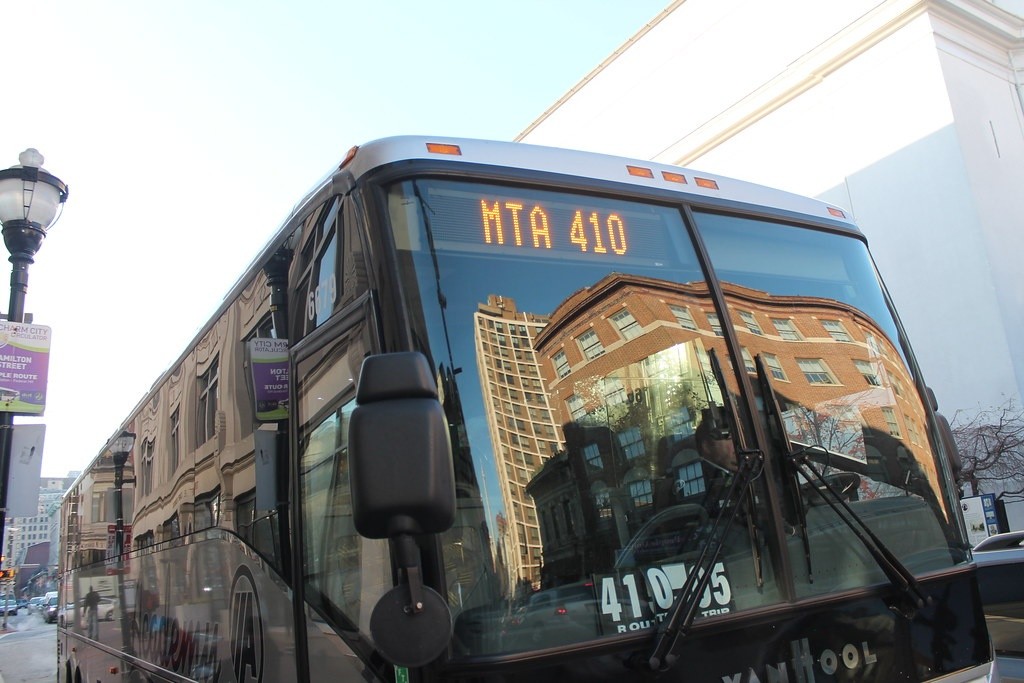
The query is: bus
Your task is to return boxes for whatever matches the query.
[54,133,997,683]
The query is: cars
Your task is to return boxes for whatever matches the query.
[514,582,647,646]
[18,599,27,609]
[0,599,19,616]
[59,598,118,629]
[27,591,58,615]
[41,597,58,623]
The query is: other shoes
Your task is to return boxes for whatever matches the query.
[87,636,92,639]
[93,636,99,641]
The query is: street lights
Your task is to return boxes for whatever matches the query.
[109,431,136,683]
[0,148,70,568]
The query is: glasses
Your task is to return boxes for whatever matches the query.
[706,428,730,440]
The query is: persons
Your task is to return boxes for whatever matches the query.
[82,586,101,641]
[695,418,739,519]
